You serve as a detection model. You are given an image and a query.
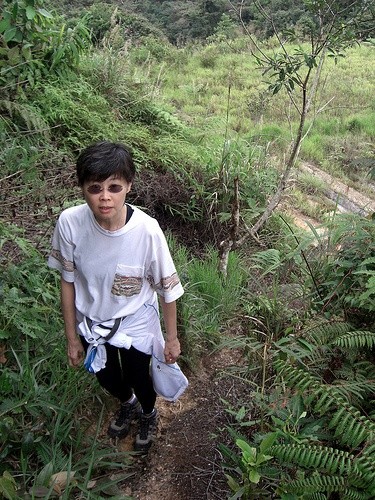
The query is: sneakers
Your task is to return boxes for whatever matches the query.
[135,408,162,454]
[107,398,140,440]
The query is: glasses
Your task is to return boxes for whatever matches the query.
[81,181,130,194]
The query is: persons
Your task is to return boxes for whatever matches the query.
[45,140,185,456]
